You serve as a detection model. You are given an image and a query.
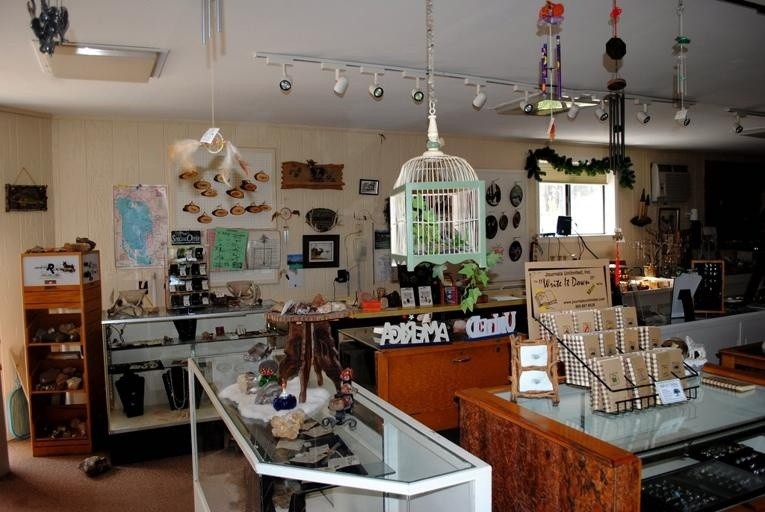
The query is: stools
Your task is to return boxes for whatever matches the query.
[264,308,349,404]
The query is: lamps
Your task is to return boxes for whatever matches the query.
[278,53,692,126]
[495,2,602,118]
[27,0,69,54]
[730,113,745,134]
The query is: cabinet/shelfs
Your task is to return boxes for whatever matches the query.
[633,294,764,365]
[101,299,273,448]
[454,364,764,511]
[718,340,764,372]
[185,354,492,512]
[21,249,102,457]
[375,336,511,432]
[335,286,526,326]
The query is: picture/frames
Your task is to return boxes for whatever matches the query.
[359,179,380,195]
[671,273,703,322]
[302,235,340,268]
[658,208,680,233]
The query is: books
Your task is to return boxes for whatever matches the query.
[540,305,687,413]
[701,376,757,392]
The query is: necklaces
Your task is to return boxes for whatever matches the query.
[169,371,185,409]
